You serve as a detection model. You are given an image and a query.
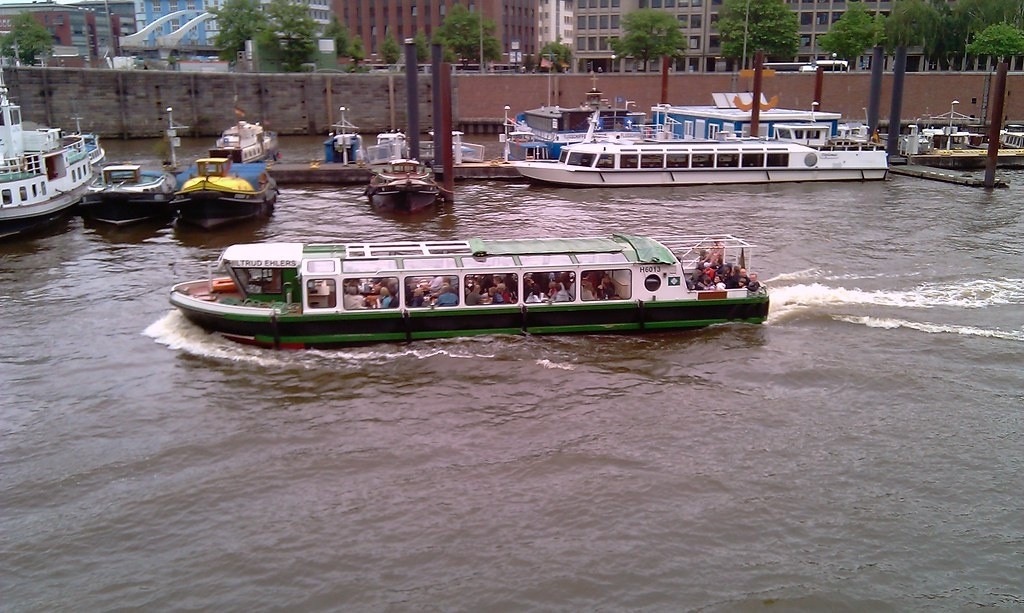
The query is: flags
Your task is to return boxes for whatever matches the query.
[235,106,245,117]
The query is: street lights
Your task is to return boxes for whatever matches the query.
[611,55,615,72]
[832,53,837,73]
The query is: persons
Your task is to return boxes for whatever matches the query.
[675,238,762,292]
[342,271,617,311]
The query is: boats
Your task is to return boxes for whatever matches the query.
[497,74,1024,185]
[78,161,177,224]
[169,233,769,351]
[0,68,106,237]
[209,121,278,164]
[364,156,439,214]
[173,158,281,228]
